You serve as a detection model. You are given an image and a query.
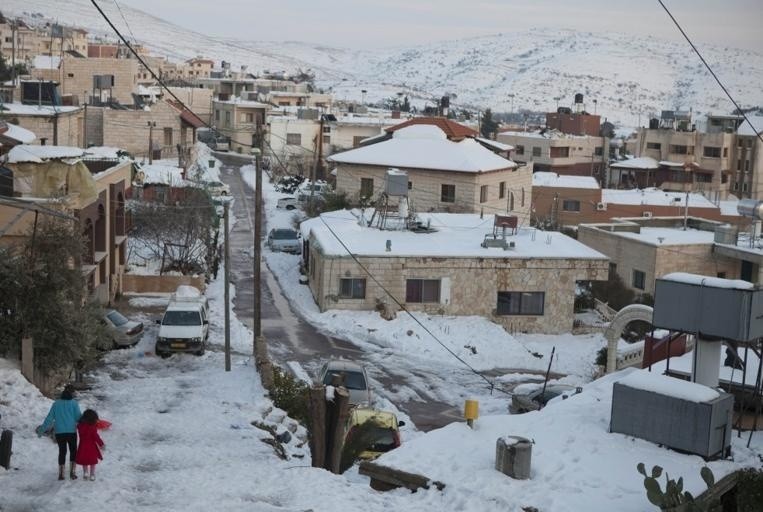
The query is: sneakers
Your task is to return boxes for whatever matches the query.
[83,473,89,479]
[58,475,66,480]
[90,475,96,481]
[70,474,78,480]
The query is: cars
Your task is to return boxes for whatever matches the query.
[197,179,231,197]
[155,293,210,359]
[207,134,229,152]
[94,308,145,353]
[340,407,406,474]
[267,227,301,256]
[512,383,576,415]
[317,359,372,409]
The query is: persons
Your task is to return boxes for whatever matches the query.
[35,388,84,481]
[73,408,104,481]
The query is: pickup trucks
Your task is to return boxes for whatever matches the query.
[276,193,320,210]
